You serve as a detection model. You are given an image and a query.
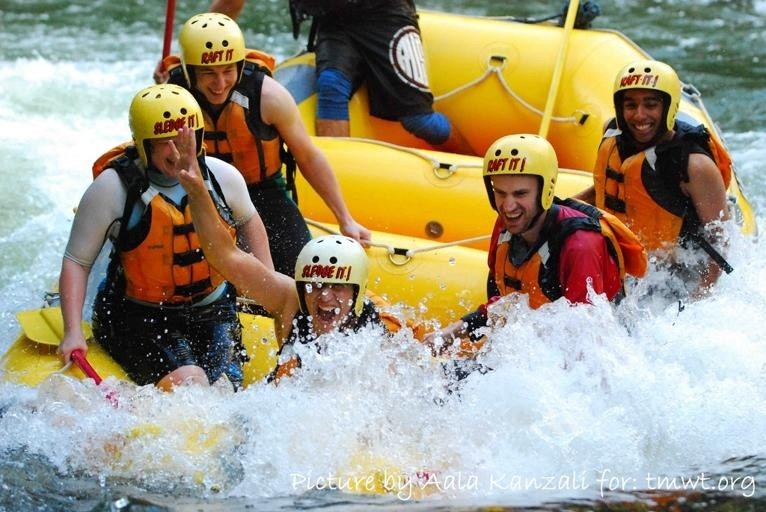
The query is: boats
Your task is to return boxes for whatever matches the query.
[2,7,759,512]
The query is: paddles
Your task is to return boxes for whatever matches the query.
[16,305,117,406]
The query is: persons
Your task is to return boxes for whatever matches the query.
[165,125,426,382]
[58,82,278,393]
[289,0,476,158]
[573,59,732,303]
[152,13,370,317]
[422,134,625,403]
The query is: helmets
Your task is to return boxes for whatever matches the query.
[483,134,558,211]
[294,235,371,318]
[129,84,206,168]
[179,13,246,88]
[612,60,681,135]
